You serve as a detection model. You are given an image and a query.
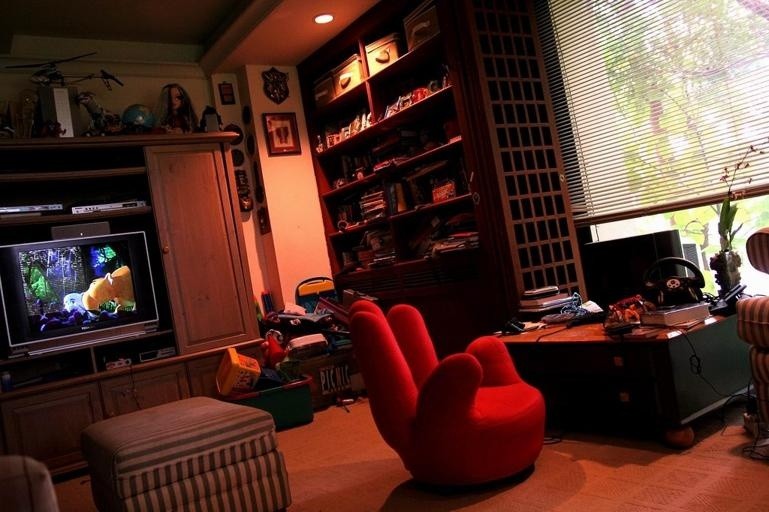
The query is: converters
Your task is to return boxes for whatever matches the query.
[746,394,759,414]
[606,322,632,337]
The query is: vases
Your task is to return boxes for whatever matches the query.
[709,252,742,297]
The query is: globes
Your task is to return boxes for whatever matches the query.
[123,104,155,129]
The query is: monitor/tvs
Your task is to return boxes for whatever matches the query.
[0,230,159,356]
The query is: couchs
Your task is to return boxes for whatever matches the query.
[349,299,547,485]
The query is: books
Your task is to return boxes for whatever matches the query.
[622,303,713,339]
[519,293,573,313]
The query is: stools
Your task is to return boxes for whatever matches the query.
[81,396,299,512]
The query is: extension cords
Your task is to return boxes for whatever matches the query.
[743,412,765,437]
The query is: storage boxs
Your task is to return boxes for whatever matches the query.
[295,350,353,414]
[228,374,316,433]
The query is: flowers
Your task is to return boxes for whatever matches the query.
[706,143,767,252]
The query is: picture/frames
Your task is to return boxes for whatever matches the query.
[259,112,303,156]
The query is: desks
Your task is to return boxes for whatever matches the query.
[489,302,753,454]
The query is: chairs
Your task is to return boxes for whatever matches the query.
[735,227,769,460]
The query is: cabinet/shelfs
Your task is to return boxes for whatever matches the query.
[297,1,488,269]
[101,362,189,431]
[143,141,260,354]
[1,378,101,481]
[335,251,497,362]
[0,134,149,222]
[189,342,263,405]
